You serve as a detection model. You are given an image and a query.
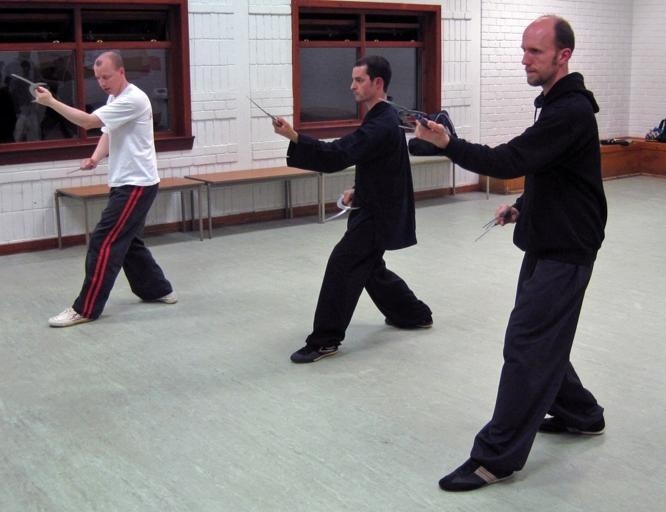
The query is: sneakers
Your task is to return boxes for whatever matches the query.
[48,306,98,327]
[385,313,434,327]
[439,456,515,491]
[290,344,338,362]
[142,290,177,304]
[538,415,604,435]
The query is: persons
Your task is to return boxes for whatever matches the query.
[33,51,177,327]
[413,12,609,494]
[271,54,435,364]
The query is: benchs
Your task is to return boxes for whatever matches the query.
[51,171,209,254]
[317,154,491,224]
[184,166,331,241]
[478,132,665,195]
